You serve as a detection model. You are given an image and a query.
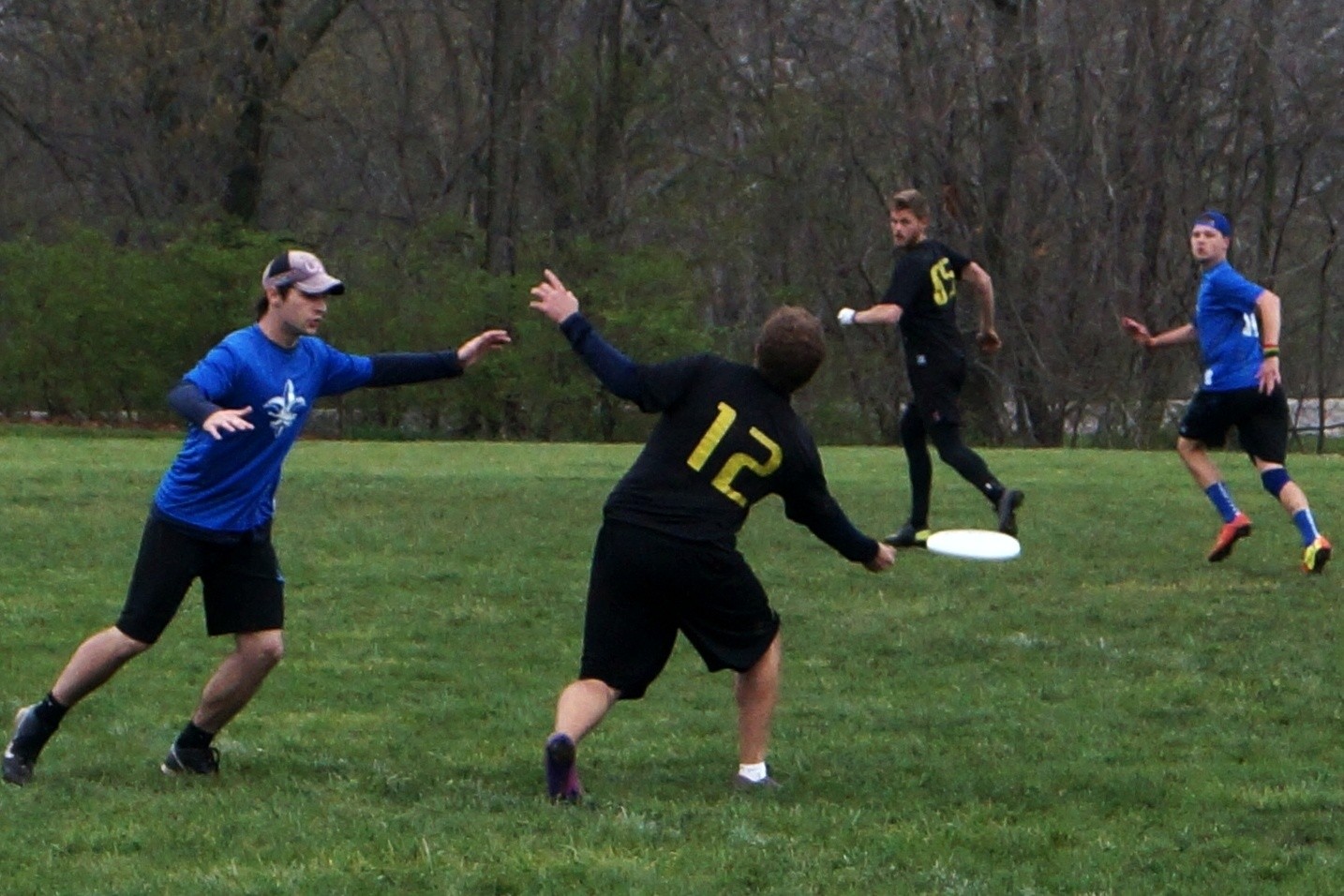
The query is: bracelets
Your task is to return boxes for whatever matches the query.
[1262,344,1281,357]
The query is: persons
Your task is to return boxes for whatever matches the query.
[530,267,896,802]
[837,189,1024,551]
[1123,211,1333,572]
[1,248,509,788]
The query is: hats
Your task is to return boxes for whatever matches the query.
[261,250,345,296]
[1194,209,1231,236]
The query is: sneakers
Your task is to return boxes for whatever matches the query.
[731,765,783,799]
[991,490,1025,537]
[1209,513,1252,563]
[2,703,50,791]
[158,741,224,790]
[881,515,930,553]
[1301,533,1333,576]
[545,732,584,807]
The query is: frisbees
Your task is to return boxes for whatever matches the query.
[926,529,1021,561]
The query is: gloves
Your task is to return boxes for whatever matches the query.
[837,307,858,326]
